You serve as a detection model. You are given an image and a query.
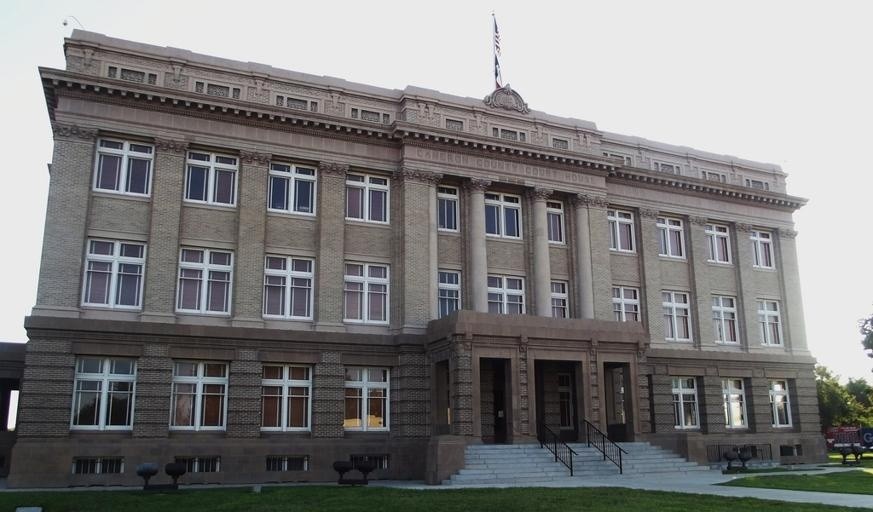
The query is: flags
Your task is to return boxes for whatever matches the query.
[490,12,506,94]
